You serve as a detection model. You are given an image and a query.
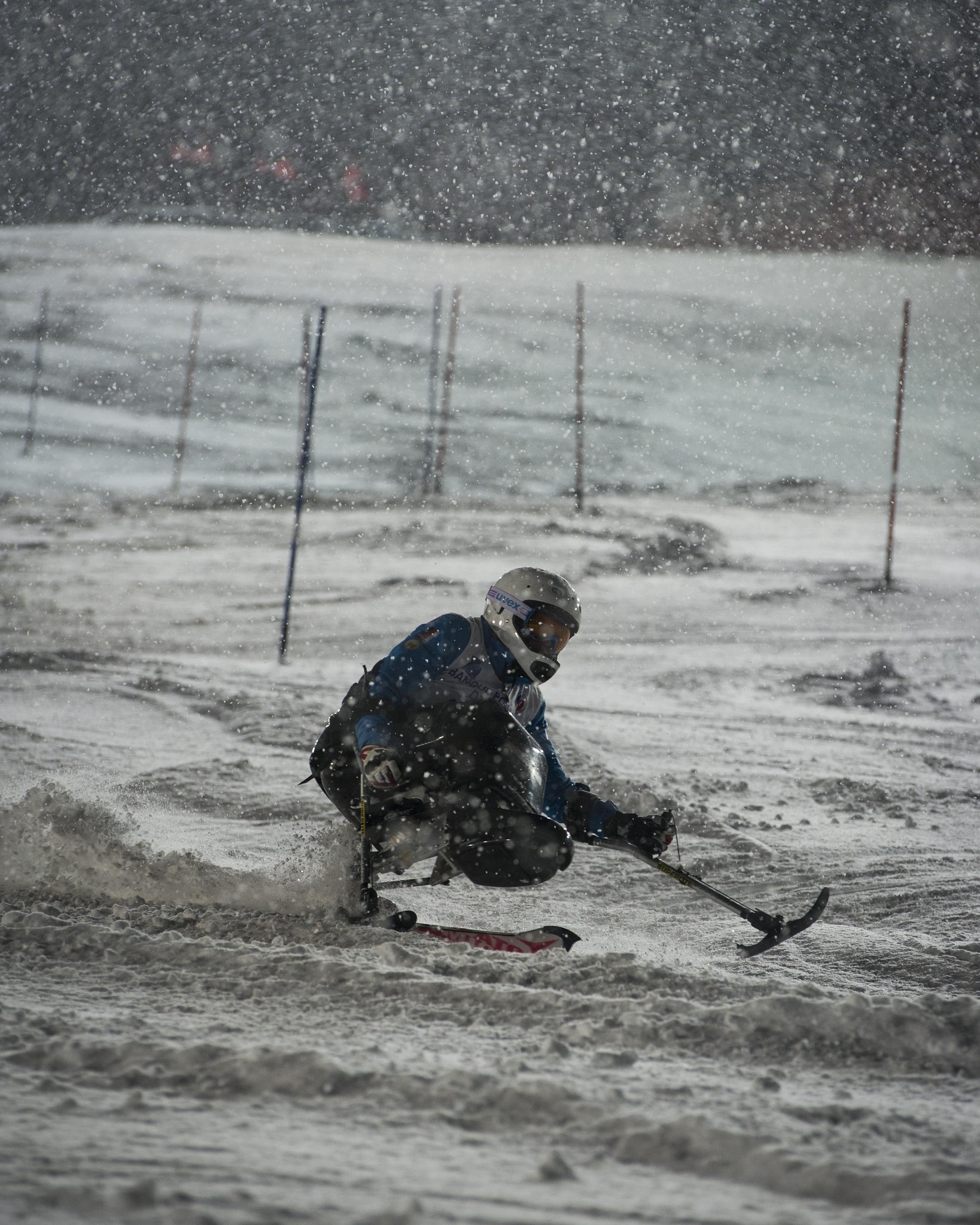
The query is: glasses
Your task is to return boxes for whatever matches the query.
[524,607,572,654]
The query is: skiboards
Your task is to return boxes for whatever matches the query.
[339,907,582,953]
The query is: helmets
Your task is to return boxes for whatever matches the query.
[485,566,581,682]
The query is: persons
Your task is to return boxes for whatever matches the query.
[311,565,676,926]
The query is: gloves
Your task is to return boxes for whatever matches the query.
[361,742,405,790]
[615,812,676,859]
